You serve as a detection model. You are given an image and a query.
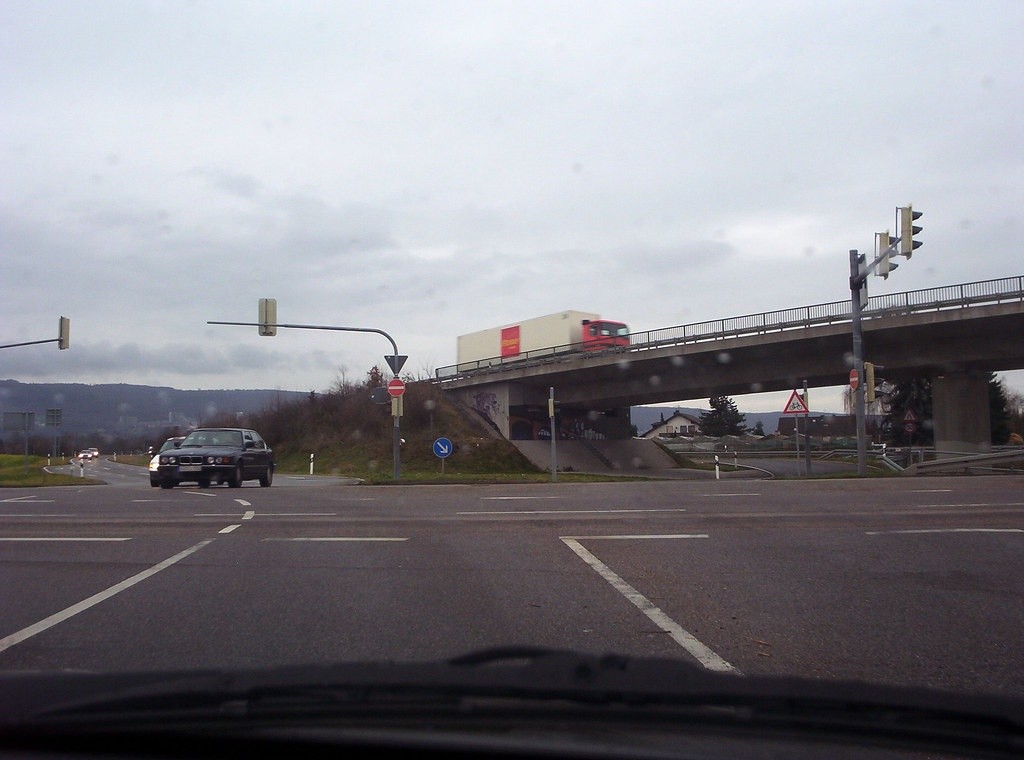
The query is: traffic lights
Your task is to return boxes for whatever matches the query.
[877,232,898,279]
[902,204,923,261]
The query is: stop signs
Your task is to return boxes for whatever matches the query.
[848,369,859,390]
[388,380,406,397]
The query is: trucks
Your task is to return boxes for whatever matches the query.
[456,309,632,379]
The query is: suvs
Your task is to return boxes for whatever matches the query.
[148,436,185,489]
[157,427,277,489]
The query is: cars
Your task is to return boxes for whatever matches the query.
[88,447,100,459]
[78,450,92,461]
